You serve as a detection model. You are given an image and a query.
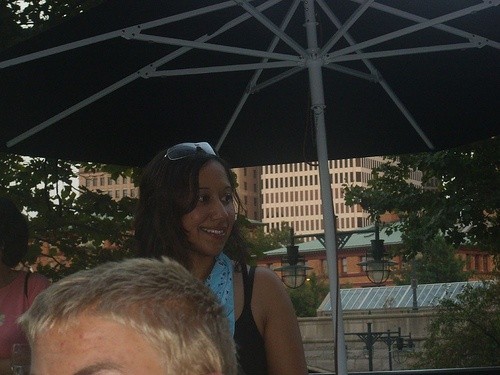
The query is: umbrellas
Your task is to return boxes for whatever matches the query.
[0,0,500,375]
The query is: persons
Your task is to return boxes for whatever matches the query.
[129,142,309,375]
[16,255,242,375]
[0,194,52,375]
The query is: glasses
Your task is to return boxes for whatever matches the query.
[163,142,217,161]
